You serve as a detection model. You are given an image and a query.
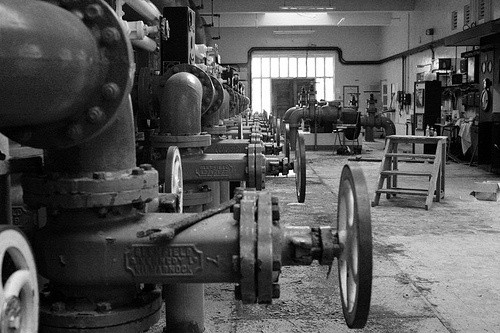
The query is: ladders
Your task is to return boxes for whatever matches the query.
[371,135,449,209]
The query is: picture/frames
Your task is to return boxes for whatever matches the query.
[343,85,359,108]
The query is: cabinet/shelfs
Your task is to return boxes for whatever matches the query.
[413,81,442,160]
[478,120,500,175]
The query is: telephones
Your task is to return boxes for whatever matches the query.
[404,93,411,105]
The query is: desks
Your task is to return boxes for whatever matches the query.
[333,125,360,156]
[435,123,480,168]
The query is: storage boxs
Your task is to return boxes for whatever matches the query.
[470,181,500,201]
